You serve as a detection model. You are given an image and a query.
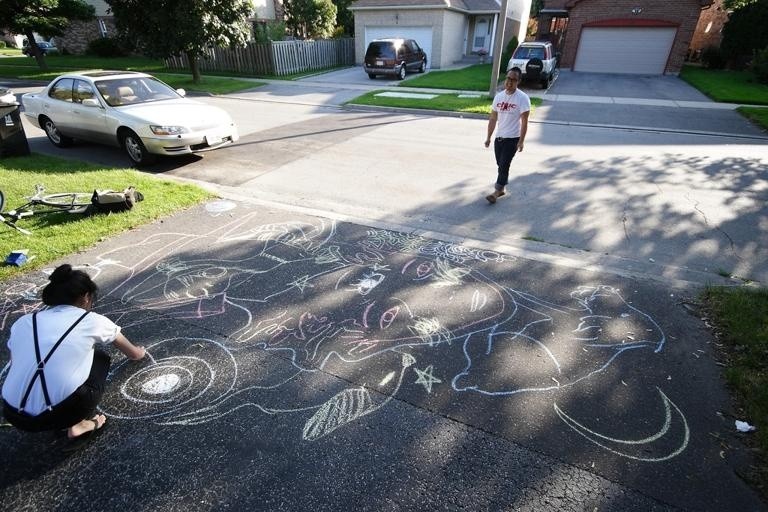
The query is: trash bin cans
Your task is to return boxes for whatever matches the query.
[91,39,111,58]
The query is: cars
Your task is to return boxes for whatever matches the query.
[22,70,240,163]
[22,41,58,58]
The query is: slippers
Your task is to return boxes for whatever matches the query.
[60,411,107,448]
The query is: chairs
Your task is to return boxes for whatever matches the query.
[114,86,142,105]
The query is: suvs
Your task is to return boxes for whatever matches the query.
[506,40,557,89]
[363,37,426,81]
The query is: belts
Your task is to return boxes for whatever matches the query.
[495,137,516,144]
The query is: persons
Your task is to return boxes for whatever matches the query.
[0,263,149,454]
[484,64,531,204]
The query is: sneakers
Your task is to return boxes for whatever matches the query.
[485,192,506,203]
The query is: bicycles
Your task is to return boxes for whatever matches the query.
[0,184,144,235]
